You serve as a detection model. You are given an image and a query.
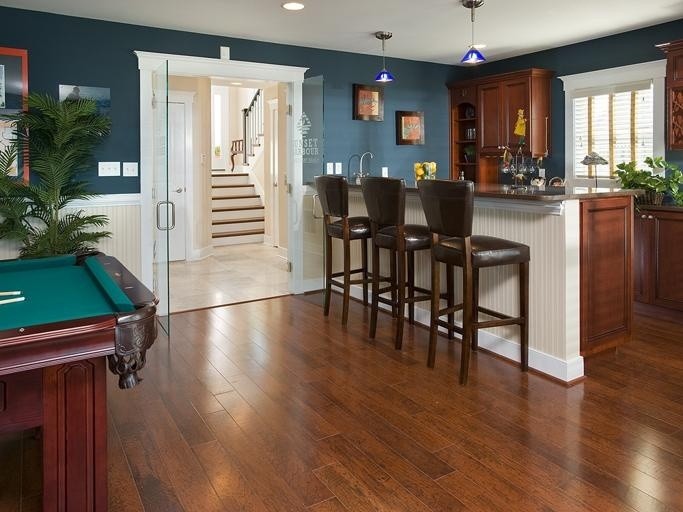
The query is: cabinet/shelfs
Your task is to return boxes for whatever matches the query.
[444,68,556,184]
[655,38,683,152]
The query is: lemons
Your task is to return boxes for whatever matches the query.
[414,161,437,181]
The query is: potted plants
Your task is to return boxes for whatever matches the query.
[612,157,683,213]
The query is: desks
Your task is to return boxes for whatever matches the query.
[0,245,159,512]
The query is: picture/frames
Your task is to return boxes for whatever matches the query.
[352,83,385,121]
[395,111,425,146]
[0,47,30,187]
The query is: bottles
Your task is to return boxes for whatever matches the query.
[458,170,465,181]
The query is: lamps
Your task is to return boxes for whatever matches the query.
[579,152,608,187]
[375,31,394,82]
[462,0,486,64]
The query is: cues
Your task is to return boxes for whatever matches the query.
[1,291,26,304]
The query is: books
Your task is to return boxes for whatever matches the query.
[466,128,476,140]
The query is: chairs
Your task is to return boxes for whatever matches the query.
[360,176,455,351]
[230,139,243,172]
[417,179,530,385]
[314,175,396,328]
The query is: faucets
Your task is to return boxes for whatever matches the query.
[353,151,373,178]
